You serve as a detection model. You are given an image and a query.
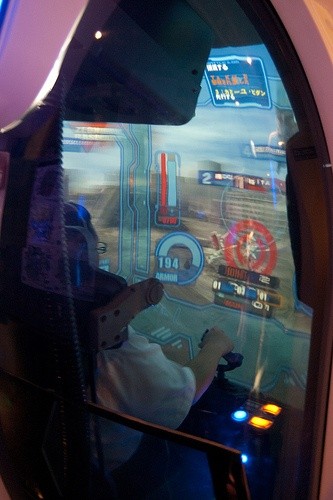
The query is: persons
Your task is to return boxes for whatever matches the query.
[45,203,237,500]
